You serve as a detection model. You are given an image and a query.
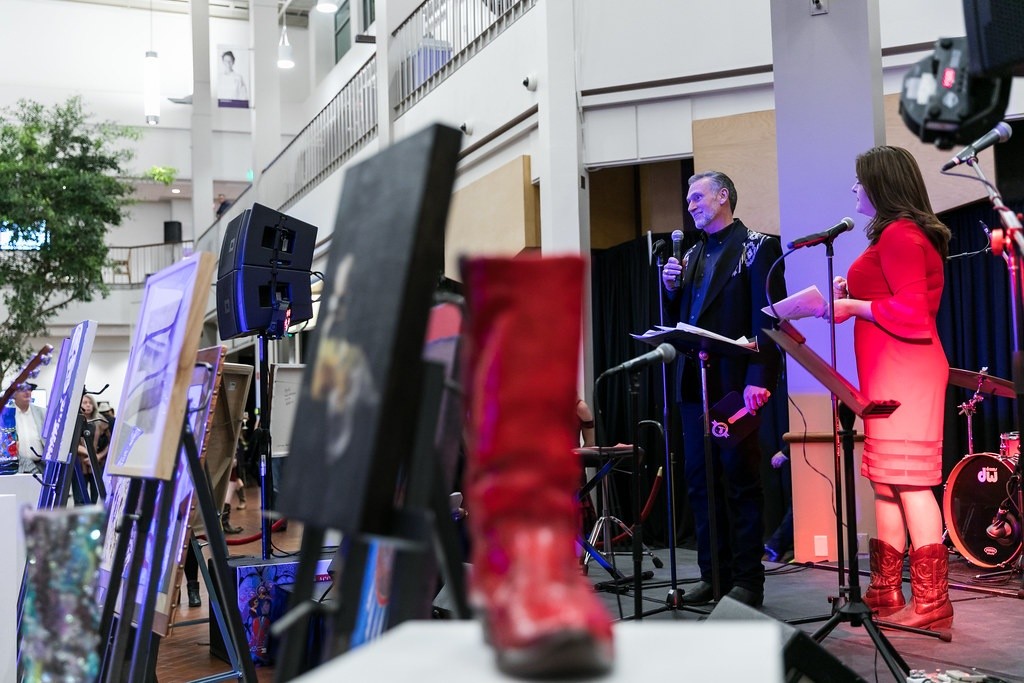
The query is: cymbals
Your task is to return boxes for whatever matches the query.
[948,368,1018,399]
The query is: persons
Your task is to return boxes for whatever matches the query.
[816,146,953,631]
[661,172,786,609]
[223,458,247,535]
[14,389,47,474]
[78,393,116,504]
[218,51,247,100]
[575,398,597,537]
[176,538,201,607]
[216,194,230,219]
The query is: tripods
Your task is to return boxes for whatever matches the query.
[763,243,953,682]
[612,239,714,621]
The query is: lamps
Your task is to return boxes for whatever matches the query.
[277,12,295,69]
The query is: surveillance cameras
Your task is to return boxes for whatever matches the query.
[523,76,536,91]
[460,122,472,135]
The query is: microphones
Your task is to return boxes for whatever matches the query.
[980,221,1009,264]
[602,342,676,378]
[787,217,855,249]
[986,521,1011,539]
[672,230,684,288]
[941,122,1012,171]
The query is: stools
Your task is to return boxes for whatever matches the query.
[573,446,663,576]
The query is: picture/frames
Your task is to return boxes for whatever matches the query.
[106,252,215,482]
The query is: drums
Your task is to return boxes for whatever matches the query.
[999,432,1022,458]
[942,452,1023,569]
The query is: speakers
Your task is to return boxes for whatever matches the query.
[963,0,1024,77]
[703,596,869,683]
[164,221,182,243]
[216,202,318,341]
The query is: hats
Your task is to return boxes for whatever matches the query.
[98,403,110,413]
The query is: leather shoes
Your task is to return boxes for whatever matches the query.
[681,580,714,605]
[727,586,763,607]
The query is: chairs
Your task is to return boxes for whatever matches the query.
[111,248,133,283]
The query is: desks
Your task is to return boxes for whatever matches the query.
[292,618,787,682]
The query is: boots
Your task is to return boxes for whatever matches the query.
[222,503,243,532]
[236,484,246,509]
[873,543,953,632]
[187,582,201,606]
[461,253,611,678]
[860,538,907,618]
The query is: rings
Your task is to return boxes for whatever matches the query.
[757,402,762,405]
[665,269,668,273]
